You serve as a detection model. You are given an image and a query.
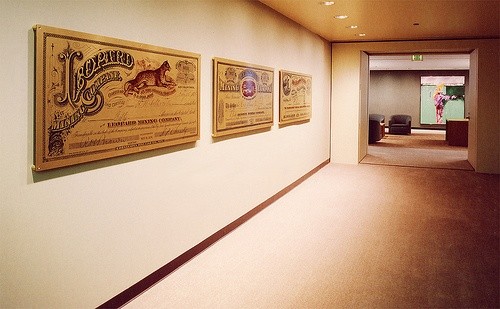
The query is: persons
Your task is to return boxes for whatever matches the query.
[433,92,464,123]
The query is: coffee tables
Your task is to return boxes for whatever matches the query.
[380,122,385,139]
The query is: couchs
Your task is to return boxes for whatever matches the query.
[389,114,412,135]
[369,120,380,144]
[369,114,384,123]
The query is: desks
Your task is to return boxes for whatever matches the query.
[446,119,469,148]
